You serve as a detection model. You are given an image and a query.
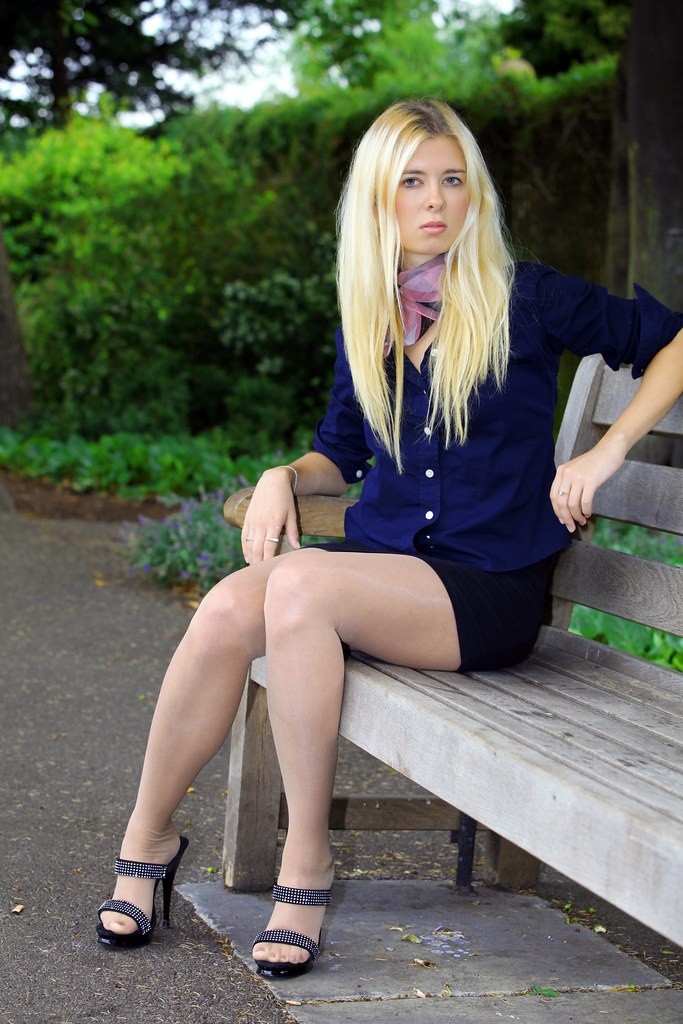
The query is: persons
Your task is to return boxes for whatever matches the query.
[96,93,682,987]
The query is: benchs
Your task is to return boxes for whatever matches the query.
[223,352,683,950]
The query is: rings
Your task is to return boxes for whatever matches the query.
[265,538,279,542]
[559,488,570,497]
[246,538,253,541]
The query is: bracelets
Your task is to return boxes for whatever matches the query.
[278,465,298,493]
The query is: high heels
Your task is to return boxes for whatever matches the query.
[96,836,188,948]
[252,880,333,979]
